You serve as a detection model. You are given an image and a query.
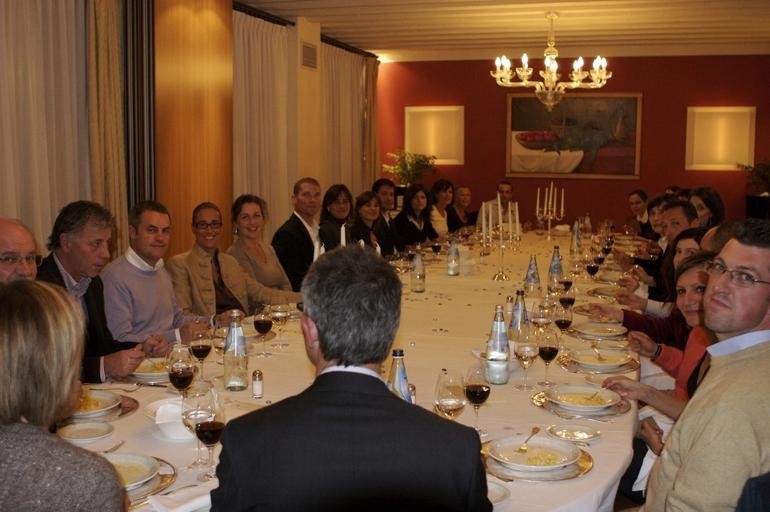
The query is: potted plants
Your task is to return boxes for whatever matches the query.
[381,151,437,212]
[735,160,770,221]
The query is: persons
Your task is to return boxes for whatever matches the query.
[35,200,169,383]
[99,202,216,349]
[319,179,518,260]
[223,193,291,292]
[166,203,305,328]
[616,219,770,512]
[612,183,724,512]
[0,270,0,283]
[270,178,333,292]
[209,245,493,512]
[2,219,42,283]
[0,278,125,512]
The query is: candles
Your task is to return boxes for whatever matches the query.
[481,201,487,248]
[542,187,549,216]
[508,201,513,240]
[535,186,541,216]
[515,201,520,238]
[560,187,565,219]
[554,186,558,215]
[548,179,554,216]
[497,190,503,233]
[488,202,492,243]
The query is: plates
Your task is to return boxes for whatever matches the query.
[70,390,122,419]
[486,235,643,487]
[126,456,175,506]
[100,452,161,493]
[56,394,139,427]
[214,326,257,340]
[143,397,183,420]
[130,357,170,383]
[57,422,114,444]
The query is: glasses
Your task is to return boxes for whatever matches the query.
[703,257,770,288]
[296,301,321,340]
[193,221,222,230]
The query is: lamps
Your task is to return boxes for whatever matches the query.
[490,10,612,113]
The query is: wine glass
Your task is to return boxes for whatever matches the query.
[434,371,465,423]
[182,384,226,484]
[253,303,290,358]
[162,329,214,405]
[512,230,615,391]
[394,257,408,287]
[465,375,491,433]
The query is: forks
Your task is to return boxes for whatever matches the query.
[516,344,608,455]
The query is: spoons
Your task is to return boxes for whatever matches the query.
[88,385,141,392]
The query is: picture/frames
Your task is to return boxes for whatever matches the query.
[505,92,643,181]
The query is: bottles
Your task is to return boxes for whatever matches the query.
[410,245,426,293]
[386,349,411,405]
[483,211,592,386]
[224,309,249,391]
[252,368,264,401]
